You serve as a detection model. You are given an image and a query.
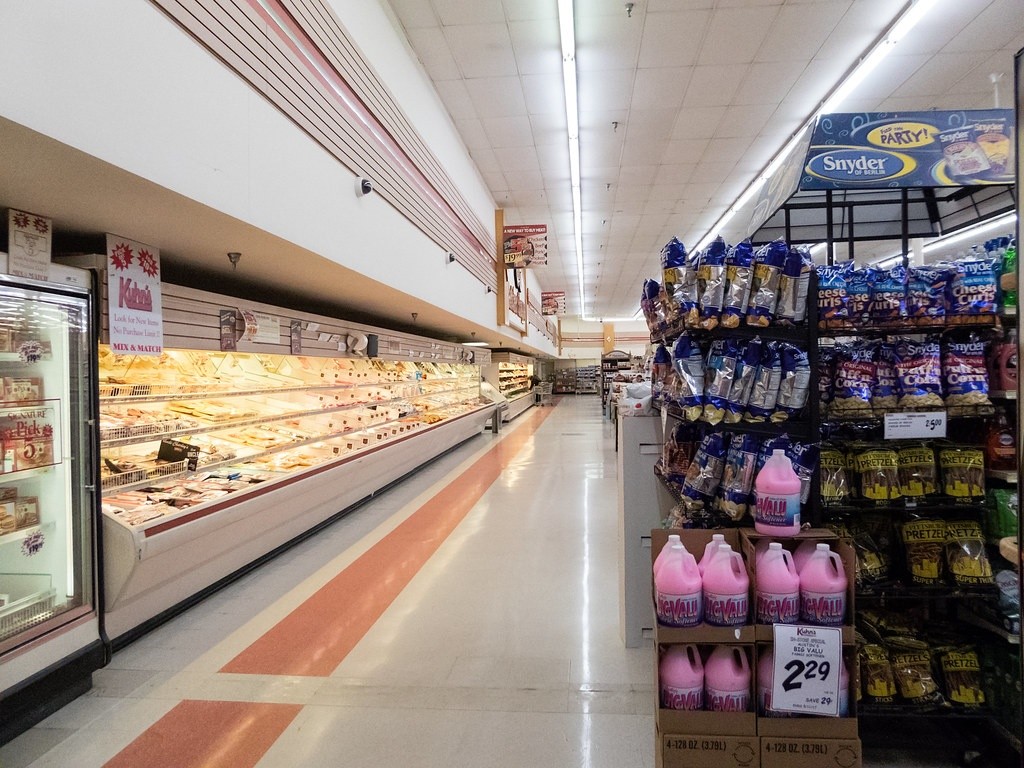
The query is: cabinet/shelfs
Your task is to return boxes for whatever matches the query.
[93,268,507,651]
[600,106,1024,768]
[491,351,538,423]
[554,365,600,394]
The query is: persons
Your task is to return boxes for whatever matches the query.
[527,375,542,402]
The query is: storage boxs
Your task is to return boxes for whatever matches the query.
[757,640,860,739]
[654,727,761,768]
[741,527,857,645]
[652,639,757,736]
[650,528,758,644]
[760,737,863,768]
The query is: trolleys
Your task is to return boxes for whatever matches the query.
[533,382,554,408]
